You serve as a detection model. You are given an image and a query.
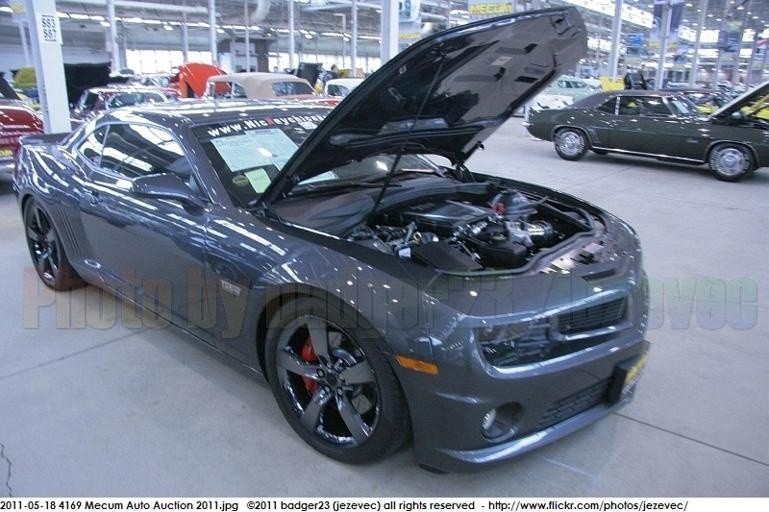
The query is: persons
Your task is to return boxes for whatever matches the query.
[272,66,279,72]
[322,65,338,95]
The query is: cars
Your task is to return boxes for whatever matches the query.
[15,6,653,474]
[523,75,769,183]
